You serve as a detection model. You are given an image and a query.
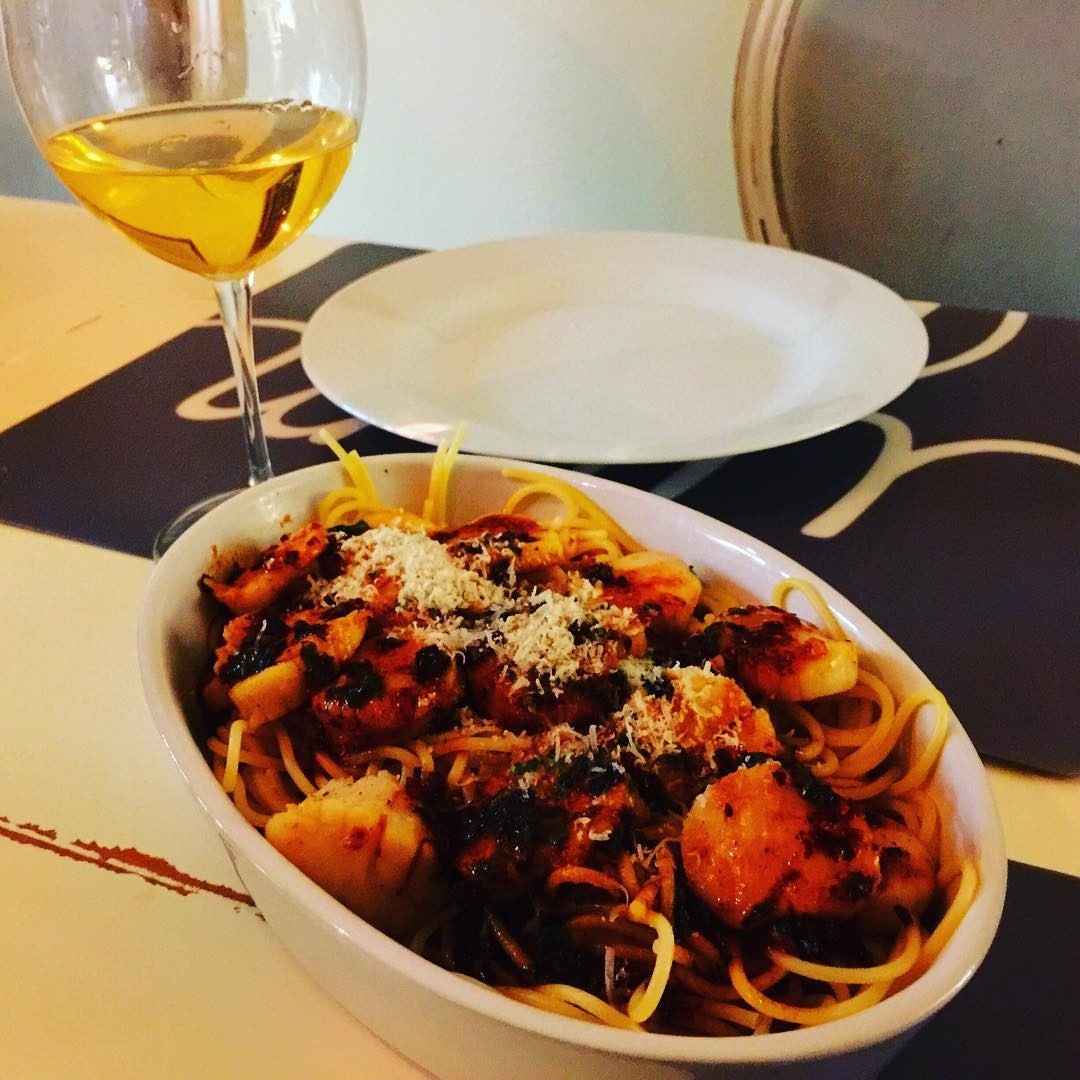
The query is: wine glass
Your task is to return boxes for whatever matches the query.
[1,0,365,564]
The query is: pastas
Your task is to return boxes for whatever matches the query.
[211,420,978,1033]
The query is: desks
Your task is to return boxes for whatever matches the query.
[1,195,1078,1080]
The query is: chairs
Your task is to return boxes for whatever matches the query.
[731,0,1080,321]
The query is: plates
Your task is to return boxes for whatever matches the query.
[297,229,929,465]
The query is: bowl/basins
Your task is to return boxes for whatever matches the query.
[133,450,1007,1078]
